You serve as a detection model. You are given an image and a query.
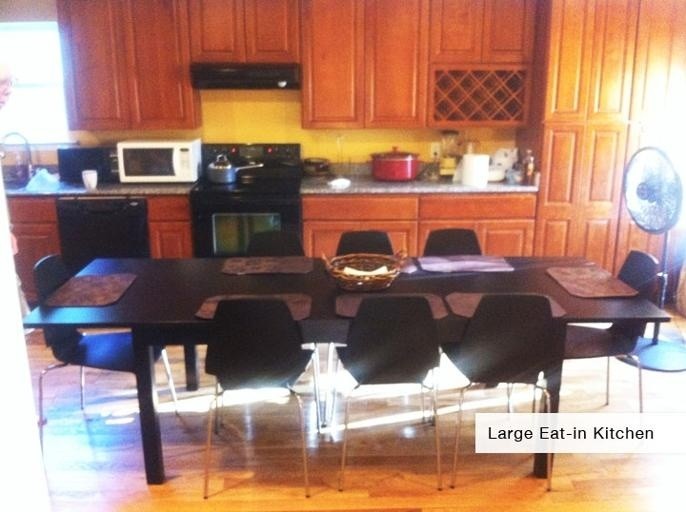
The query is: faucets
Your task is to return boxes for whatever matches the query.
[0,133,34,173]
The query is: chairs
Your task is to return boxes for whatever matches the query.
[248,230,304,257]
[564,251,659,412]
[203,298,317,499]
[335,230,394,255]
[441,294,552,493]
[329,296,441,491]
[422,229,483,256]
[34,253,178,425]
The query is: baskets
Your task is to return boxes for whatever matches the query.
[321,246,408,293]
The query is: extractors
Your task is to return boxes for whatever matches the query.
[191,63,301,90]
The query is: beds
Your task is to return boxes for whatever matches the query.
[23,257,670,484]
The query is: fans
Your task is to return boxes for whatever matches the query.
[613,146,686,373]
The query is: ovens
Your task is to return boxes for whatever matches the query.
[191,200,301,257]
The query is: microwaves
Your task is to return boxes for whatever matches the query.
[115,137,202,182]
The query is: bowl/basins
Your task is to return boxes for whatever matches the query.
[304,157,329,176]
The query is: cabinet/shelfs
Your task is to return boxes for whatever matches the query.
[302,196,536,257]
[533,0,685,279]
[188,1,302,64]
[301,2,428,127]
[430,2,533,63]
[4,197,194,310]
[56,0,206,132]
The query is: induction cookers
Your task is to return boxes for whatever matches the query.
[191,161,302,199]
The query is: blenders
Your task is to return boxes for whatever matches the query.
[436,129,460,178]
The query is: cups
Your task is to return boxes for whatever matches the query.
[81,170,97,191]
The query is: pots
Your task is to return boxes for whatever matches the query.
[207,154,264,184]
[371,145,421,181]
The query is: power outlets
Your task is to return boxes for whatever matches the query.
[431,142,440,159]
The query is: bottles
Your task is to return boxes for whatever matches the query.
[522,149,535,184]
